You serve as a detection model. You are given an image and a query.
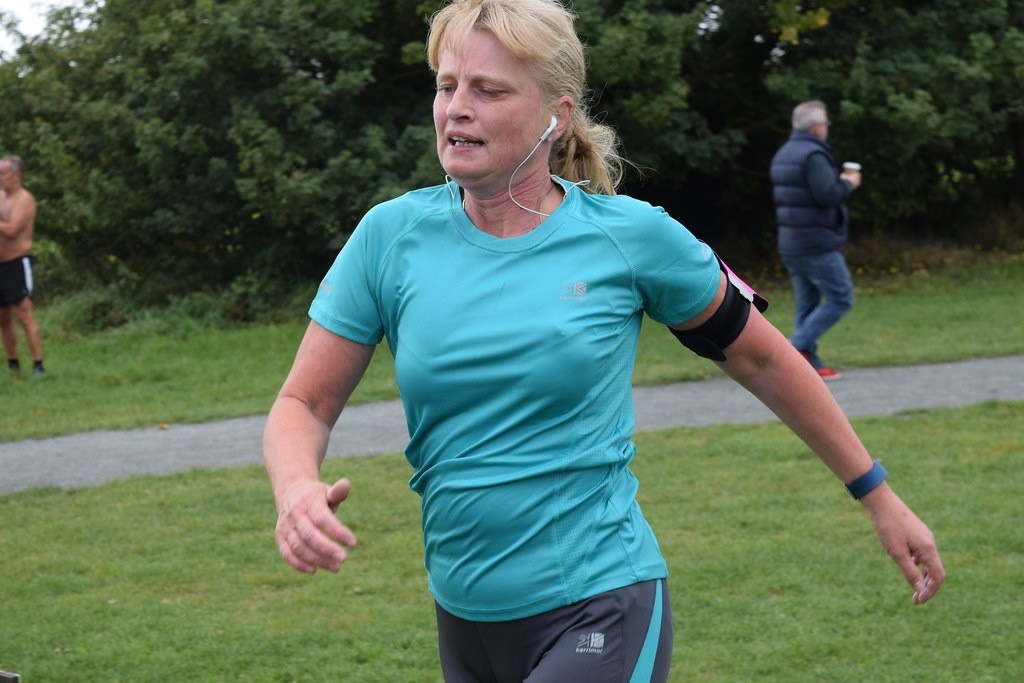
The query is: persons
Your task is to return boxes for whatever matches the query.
[0,154,48,380]
[260,0,947,682]
[769,99,862,382]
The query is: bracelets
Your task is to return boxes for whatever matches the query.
[842,459,888,500]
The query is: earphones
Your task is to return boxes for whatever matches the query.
[539,114,557,141]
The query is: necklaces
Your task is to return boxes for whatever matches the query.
[461,180,555,225]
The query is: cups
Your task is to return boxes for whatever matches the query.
[842,161,862,175]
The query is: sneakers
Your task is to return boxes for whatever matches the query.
[815,367,842,382]
[797,350,811,364]
[33,366,47,377]
